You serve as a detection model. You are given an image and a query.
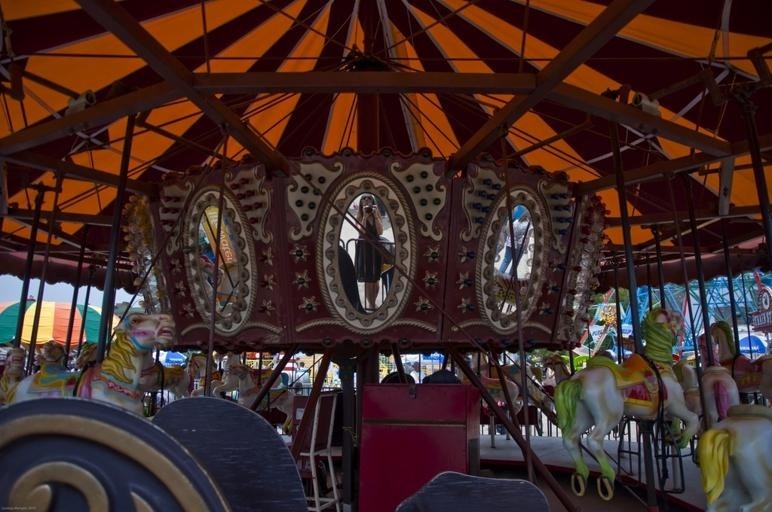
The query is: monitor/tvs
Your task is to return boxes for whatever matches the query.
[363,204,374,215]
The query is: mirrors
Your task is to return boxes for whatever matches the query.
[477,184,552,336]
[317,172,416,338]
[182,184,260,336]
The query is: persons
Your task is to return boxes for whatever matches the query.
[502,213,532,274]
[295,361,310,390]
[409,362,427,383]
[353,196,384,312]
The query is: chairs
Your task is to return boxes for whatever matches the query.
[290,393,346,511]
